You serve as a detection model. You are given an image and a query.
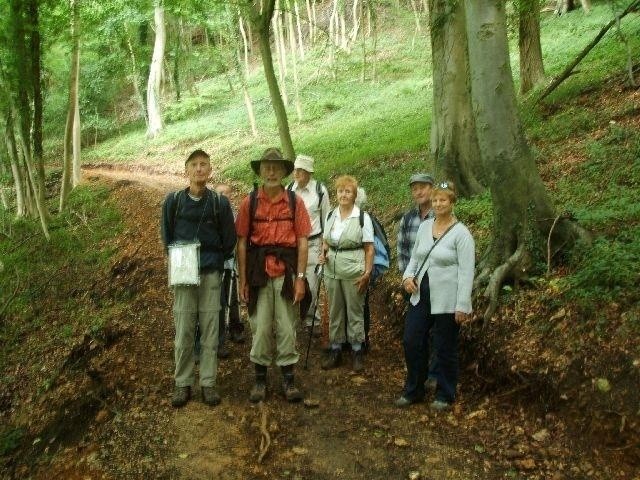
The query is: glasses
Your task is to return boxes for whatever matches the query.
[433,182,448,190]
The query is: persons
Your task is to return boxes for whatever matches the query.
[215,182,246,358]
[236,147,312,403]
[283,152,332,338]
[396,174,440,388]
[318,176,375,373]
[395,181,476,412]
[342,186,373,352]
[159,146,237,407]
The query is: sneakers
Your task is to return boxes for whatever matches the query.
[202,386,220,404]
[308,325,321,338]
[394,397,411,406]
[217,329,244,357]
[430,399,450,410]
[172,386,191,406]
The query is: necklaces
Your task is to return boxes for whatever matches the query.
[436,231,442,236]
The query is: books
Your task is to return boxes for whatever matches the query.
[170,247,198,286]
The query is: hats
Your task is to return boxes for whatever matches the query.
[251,147,294,178]
[294,155,314,172]
[408,174,434,187]
[184,148,210,166]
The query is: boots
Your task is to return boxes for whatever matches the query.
[280,373,300,400]
[350,349,363,370]
[250,374,267,400]
[323,347,343,369]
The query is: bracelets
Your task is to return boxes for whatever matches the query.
[365,271,370,275]
[297,272,304,280]
